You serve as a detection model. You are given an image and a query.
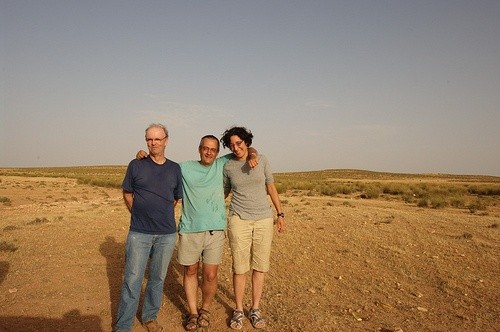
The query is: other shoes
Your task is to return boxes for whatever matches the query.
[143,320,163,332]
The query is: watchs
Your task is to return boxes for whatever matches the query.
[277,212,285,218]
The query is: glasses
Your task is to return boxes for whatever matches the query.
[229,140,243,148]
[146,137,166,143]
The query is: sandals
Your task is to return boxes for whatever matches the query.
[230,309,244,329]
[248,308,265,328]
[186,314,199,331]
[198,309,210,328]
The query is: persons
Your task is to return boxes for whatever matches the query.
[135,135,260,331]
[114,122,183,332]
[220,126,286,329]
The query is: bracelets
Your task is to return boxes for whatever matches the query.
[249,153,256,157]
